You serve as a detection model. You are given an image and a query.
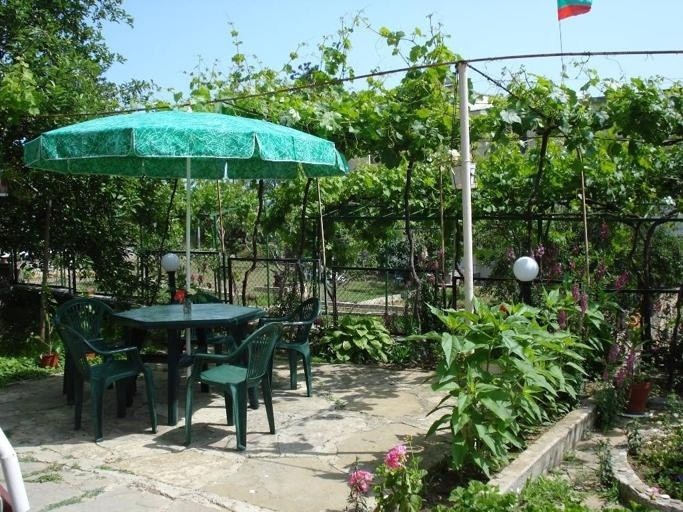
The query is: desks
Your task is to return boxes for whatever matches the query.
[111,303,263,428]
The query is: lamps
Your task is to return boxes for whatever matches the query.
[512,256,542,311]
[160,251,181,304]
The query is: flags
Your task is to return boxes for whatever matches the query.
[556,0,592,22]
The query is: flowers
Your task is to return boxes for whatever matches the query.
[339,432,430,512]
[608,310,659,383]
[174,284,199,314]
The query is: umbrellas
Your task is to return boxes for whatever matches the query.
[21,109,345,258]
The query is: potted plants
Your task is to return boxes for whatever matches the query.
[27,332,63,369]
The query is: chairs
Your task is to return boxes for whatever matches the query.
[171,291,244,368]
[247,294,324,402]
[180,321,283,453]
[51,296,158,443]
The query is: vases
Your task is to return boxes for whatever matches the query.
[623,383,652,415]
[182,296,192,314]
